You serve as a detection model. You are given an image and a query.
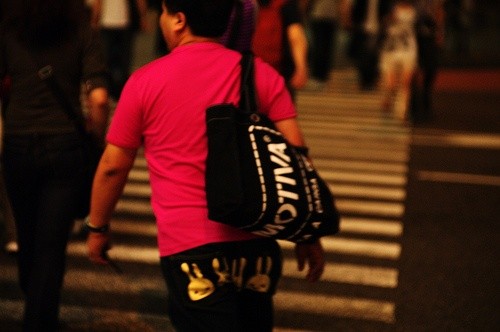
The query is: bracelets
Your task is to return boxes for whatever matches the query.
[84,213,110,233]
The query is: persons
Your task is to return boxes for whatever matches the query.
[87,0,446,124]
[2,0,111,332]
[90,0,326,331]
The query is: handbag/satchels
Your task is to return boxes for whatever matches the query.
[205,50,340,243]
